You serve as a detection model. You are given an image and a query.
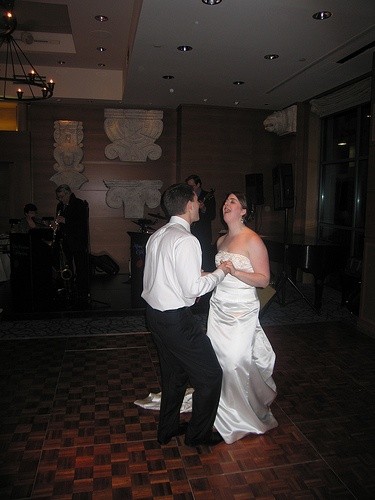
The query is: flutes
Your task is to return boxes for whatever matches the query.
[51,202,63,237]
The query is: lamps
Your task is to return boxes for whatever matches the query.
[0,0,55,101]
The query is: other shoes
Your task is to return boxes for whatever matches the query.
[183,432,224,447]
[156,421,187,444]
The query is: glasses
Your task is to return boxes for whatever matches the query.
[56,192,67,199]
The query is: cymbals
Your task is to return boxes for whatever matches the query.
[131,217,157,224]
[146,212,168,220]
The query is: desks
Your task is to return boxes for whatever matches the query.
[263,234,341,312]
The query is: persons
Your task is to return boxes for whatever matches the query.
[141,183,230,447]
[185,175,216,240]
[134,191,277,445]
[10,203,44,231]
[54,184,90,304]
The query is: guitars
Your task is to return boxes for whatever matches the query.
[200,188,217,205]
